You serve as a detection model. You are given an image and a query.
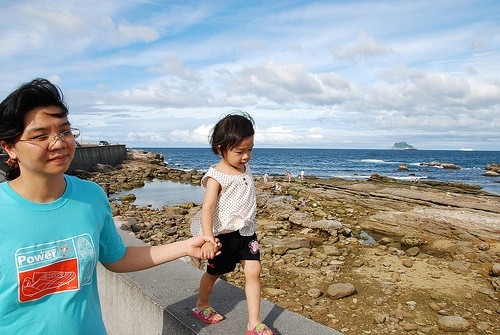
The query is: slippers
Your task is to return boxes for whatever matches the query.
[192,306,223,323]
[246,323,276,335]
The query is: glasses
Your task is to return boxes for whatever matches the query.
[18,128,80,145]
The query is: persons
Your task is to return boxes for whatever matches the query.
[190,110,276,335]
[0,78,223,335]
